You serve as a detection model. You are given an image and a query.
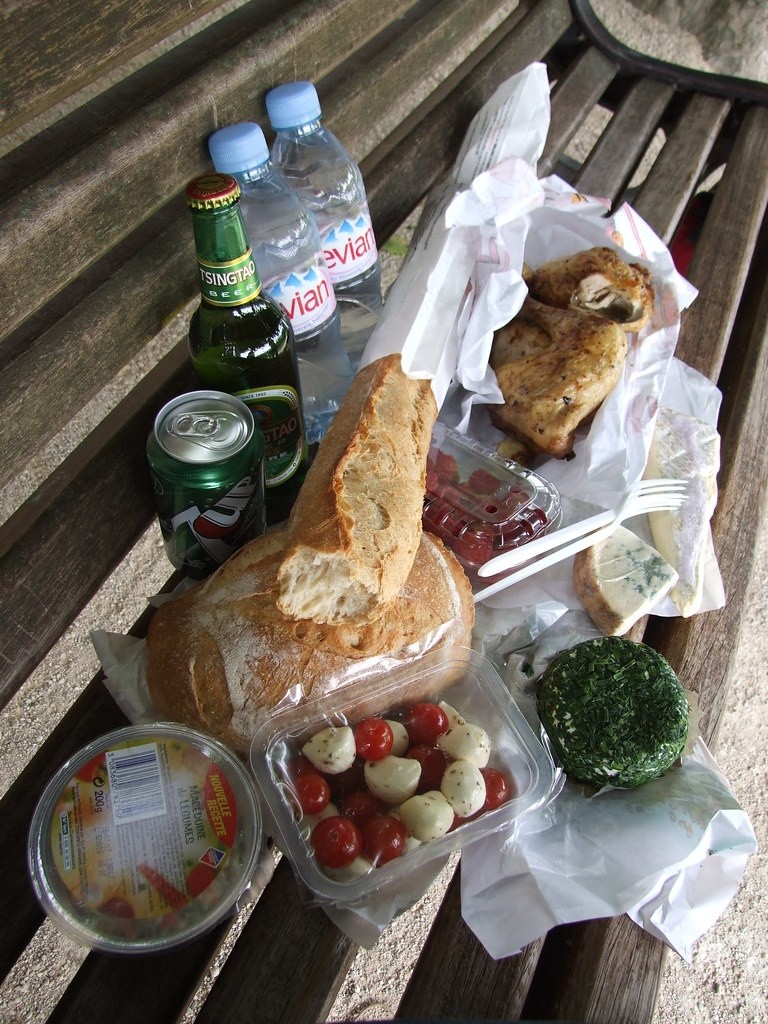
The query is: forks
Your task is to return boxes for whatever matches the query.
[473,479,689,605]
[478,485,690,578]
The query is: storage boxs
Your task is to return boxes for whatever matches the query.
[421,419,562,586]
[27,720,262,958]
[250,647,554,902]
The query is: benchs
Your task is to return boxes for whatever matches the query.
[0,0,768,1024]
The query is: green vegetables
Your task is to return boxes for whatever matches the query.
[528,637,689,788]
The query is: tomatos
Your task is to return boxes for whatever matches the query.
[290,703,512,871]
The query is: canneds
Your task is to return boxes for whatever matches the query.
[144,390,267,580]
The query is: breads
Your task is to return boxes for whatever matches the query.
[639,406,721,617]
[572,522,678,637]
[275,354,436,625]
[142,517,474,754]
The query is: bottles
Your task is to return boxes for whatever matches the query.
[209,122,353,444]
[184,175,311,513]
[265,80,385,374]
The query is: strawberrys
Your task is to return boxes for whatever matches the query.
[420,445,546,568]
